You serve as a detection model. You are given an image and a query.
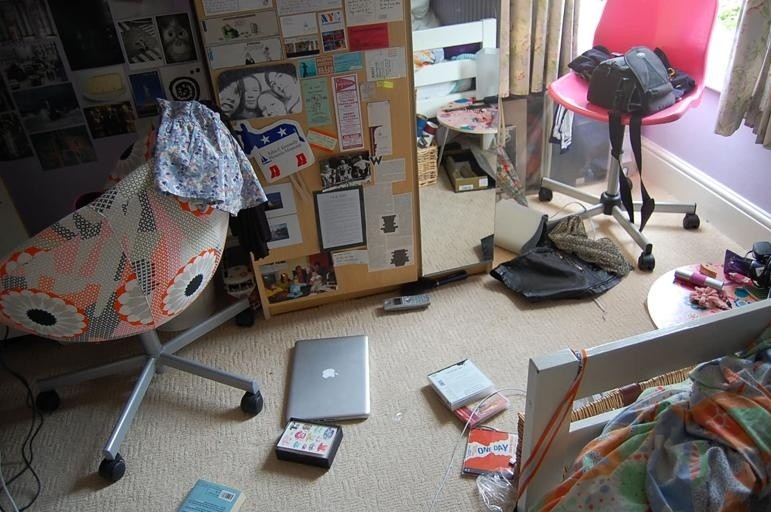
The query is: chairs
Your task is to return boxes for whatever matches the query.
[536,0,722,276]
[1,106,267,481]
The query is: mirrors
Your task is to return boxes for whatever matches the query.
[408,0,502,279]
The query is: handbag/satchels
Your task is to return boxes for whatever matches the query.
[587,46,676,114]
[654,46,696,98]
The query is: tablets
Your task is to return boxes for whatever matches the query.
[286,335,371,424]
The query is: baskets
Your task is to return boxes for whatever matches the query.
[416,141,439,186]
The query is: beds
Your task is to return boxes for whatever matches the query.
[411,18,499,114]
[518,298,770,511]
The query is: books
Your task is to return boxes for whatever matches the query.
[180,478,247,512]
[462,428,520,477]
[453,391,511,428]
[427,358,494,413]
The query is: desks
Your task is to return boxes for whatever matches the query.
[436,96,499,169]
[644,260,766,329]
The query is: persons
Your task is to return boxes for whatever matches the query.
[265,65,302,114]
[320,155,371,190]
[215,71,247,120]
[263,261,337,304]
[90,102,137,136]
[256,90,288,117]
[235,75,263,119]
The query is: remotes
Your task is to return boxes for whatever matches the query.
[385,293,432,312]
